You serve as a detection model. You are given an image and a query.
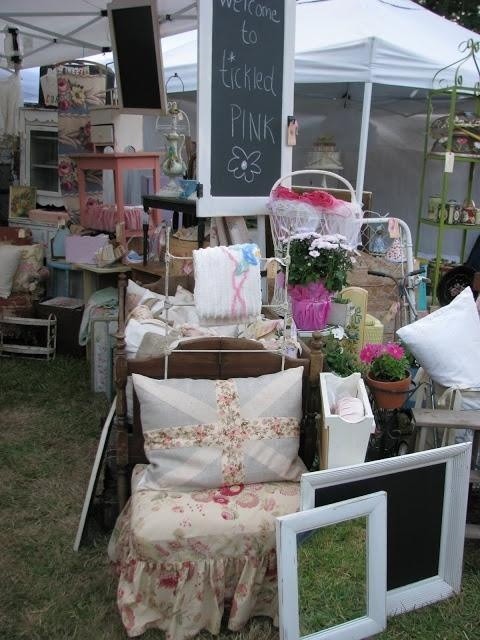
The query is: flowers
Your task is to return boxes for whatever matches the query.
[358,338,412,378]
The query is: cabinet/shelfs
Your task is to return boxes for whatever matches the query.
[0,311,58,361]
[412,37,480,307]
[16,105,171,214]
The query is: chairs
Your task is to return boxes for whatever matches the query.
[269,167,420,333]
[113,335,311,624]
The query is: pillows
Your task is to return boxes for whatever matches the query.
[130,362,308,489]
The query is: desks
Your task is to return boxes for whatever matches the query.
[69,149,160,256]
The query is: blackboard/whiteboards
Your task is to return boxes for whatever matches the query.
[196,0,296,218]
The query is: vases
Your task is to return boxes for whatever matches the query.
[365,370,411,411]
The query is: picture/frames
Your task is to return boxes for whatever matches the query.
[300,440,470,620]
[276,487,387,639]
